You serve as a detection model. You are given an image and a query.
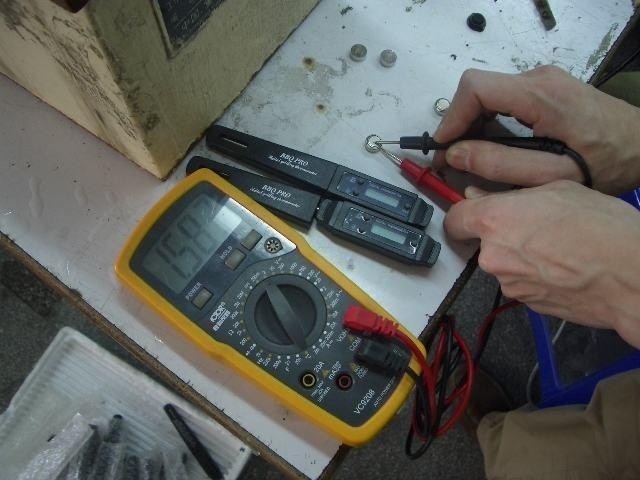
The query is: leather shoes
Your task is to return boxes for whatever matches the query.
[449,362,515,443]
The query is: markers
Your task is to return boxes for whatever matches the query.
[163,401,225,480]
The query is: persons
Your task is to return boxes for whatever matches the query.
[432,65,640,480]
